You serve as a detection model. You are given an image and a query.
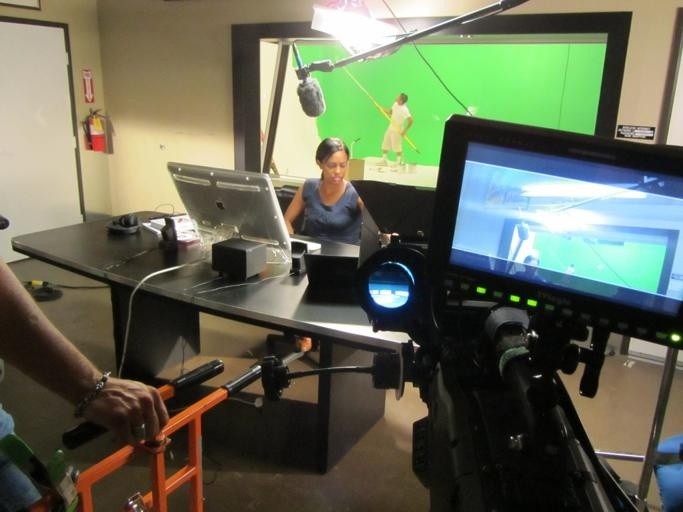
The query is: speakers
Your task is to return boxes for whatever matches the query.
[159,216,178,248]
[289,240,310,277]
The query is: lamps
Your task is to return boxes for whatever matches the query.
[299,0,400,61]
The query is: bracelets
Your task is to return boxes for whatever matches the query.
[73,370,111,418]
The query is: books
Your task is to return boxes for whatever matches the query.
[143,213,192,236]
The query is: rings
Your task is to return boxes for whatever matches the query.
[131,422,146,434]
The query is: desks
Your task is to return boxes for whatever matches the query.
[10,207,411,474]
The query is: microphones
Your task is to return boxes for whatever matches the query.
[297,75,325,119]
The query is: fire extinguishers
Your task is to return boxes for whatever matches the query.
[83,107,107,151]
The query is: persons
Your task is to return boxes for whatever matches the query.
[560,264,575,287]
[376,93,413,166]
[0,254,168,512]
[510,252,543,285]
[285,137,399,247]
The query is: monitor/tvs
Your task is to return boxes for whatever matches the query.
[423,112,683,353]
[165,160,291,253]
[253,28,613,192]
